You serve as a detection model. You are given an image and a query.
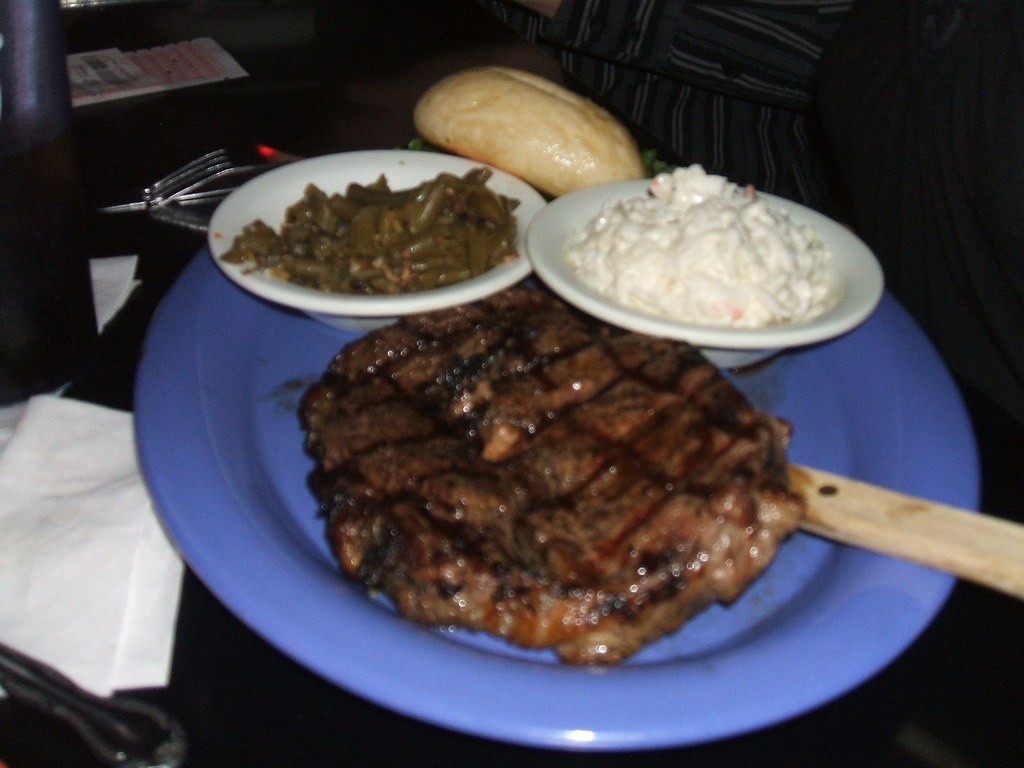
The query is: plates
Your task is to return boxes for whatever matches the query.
[132,166,985,752]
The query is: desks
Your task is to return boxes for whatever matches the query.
[0,85,1024,768]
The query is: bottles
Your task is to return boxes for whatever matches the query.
[0,0,72,149]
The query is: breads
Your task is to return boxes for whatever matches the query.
[413,65,643,197]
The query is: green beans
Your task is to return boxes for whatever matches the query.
[219,167,524,294]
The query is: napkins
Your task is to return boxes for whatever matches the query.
[0,392,185,699]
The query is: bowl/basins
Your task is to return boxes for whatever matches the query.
[522,178,884,370]
[208,147,548,329]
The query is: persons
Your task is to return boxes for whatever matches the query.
[460,1,871,236]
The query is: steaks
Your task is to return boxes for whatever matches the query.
[298,286,804,666]
